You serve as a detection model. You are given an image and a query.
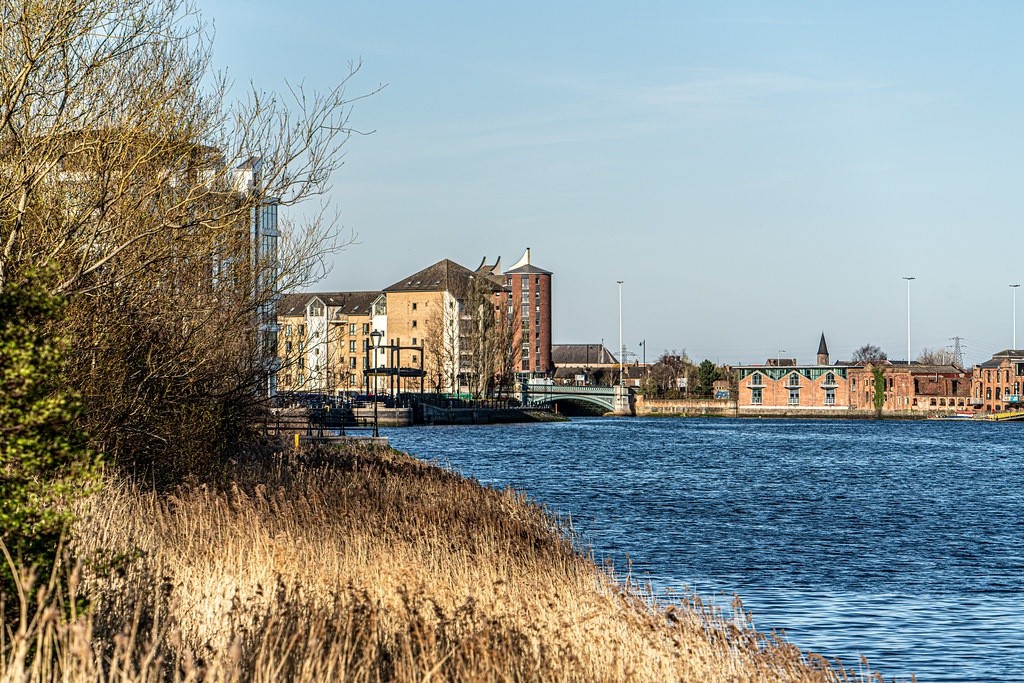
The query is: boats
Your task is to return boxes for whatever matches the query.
[269,400,329,431]
[351,398,414,428]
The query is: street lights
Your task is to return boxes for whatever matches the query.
[617,280,625,386]
[1008,283,1021,350]
[777,350,788,366]
[901,276,916,366]
[638,339,646,375]
[368,326,382,437]
